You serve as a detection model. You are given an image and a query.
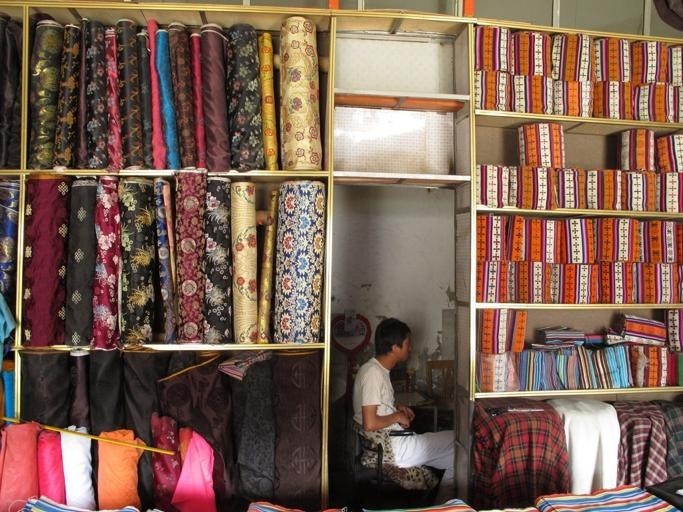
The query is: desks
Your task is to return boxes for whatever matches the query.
[392,391,438,432]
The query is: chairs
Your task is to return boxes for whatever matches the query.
[424,359,455,410]
[349,419,444,508]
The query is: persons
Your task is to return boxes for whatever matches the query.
[352,318,455,505]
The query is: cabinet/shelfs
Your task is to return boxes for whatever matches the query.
[471,16,682,492]
[0,0,25,424]
[13,2,334,511]
[328,9,475,185]
